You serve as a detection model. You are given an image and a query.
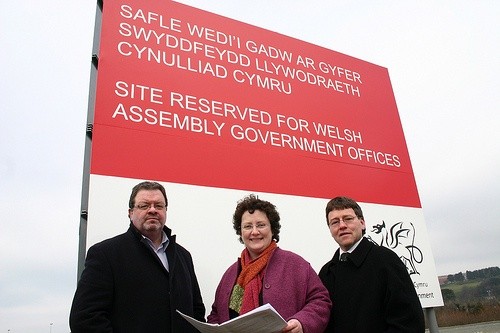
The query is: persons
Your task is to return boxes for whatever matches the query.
[318,196,425,333]
[69,182,206,333]
[207,194,332,333]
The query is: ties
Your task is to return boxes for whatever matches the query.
[341,253,348,261]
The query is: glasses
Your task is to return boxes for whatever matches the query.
[133,202,166,210]
[241,223,271,230]
[328,215,358,228]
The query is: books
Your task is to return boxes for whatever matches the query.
[176,302,288,333]
